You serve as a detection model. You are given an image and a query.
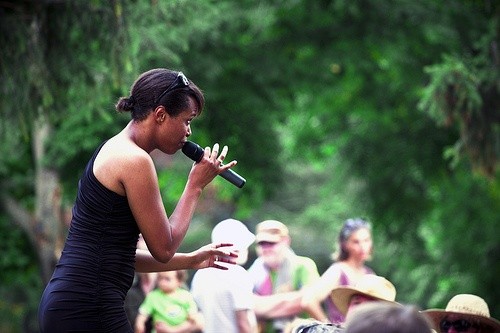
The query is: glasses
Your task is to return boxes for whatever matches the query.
[440,319,481,333]
[297,324,333,333]
[155,72,189,104]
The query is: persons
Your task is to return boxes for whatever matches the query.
[38,67,239,333]
[126,217,437,333]
[416,294,500,333]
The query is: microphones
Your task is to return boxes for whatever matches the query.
[182,140,246,188]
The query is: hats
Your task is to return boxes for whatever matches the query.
[331,274,396,316]
[420,294,500,333]
[256,219,288,244]
[211,218,256,255]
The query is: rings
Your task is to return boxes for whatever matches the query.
[215,255,219,261]
